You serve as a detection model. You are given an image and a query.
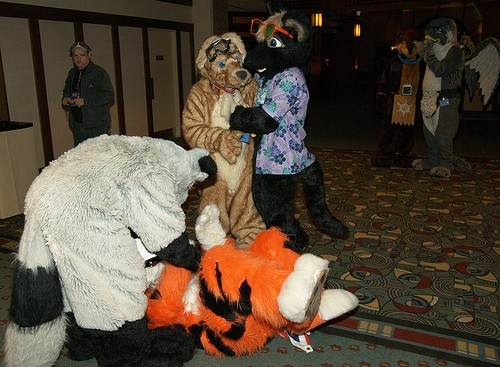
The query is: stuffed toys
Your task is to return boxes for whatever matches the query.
[142,203,359,358]
[4,134,218,367]
[370,28,427,168]
[229,9,349,249]
[182,32,266,247]
[412,17,465,177]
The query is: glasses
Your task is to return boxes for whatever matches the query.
[206,38,233,61]
[251,18,294,40]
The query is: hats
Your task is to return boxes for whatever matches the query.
[69,42,92,54]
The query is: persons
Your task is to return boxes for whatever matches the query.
[61,41,115,147]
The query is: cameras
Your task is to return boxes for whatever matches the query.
[402,84,412,95]
[65,103,82,122]
